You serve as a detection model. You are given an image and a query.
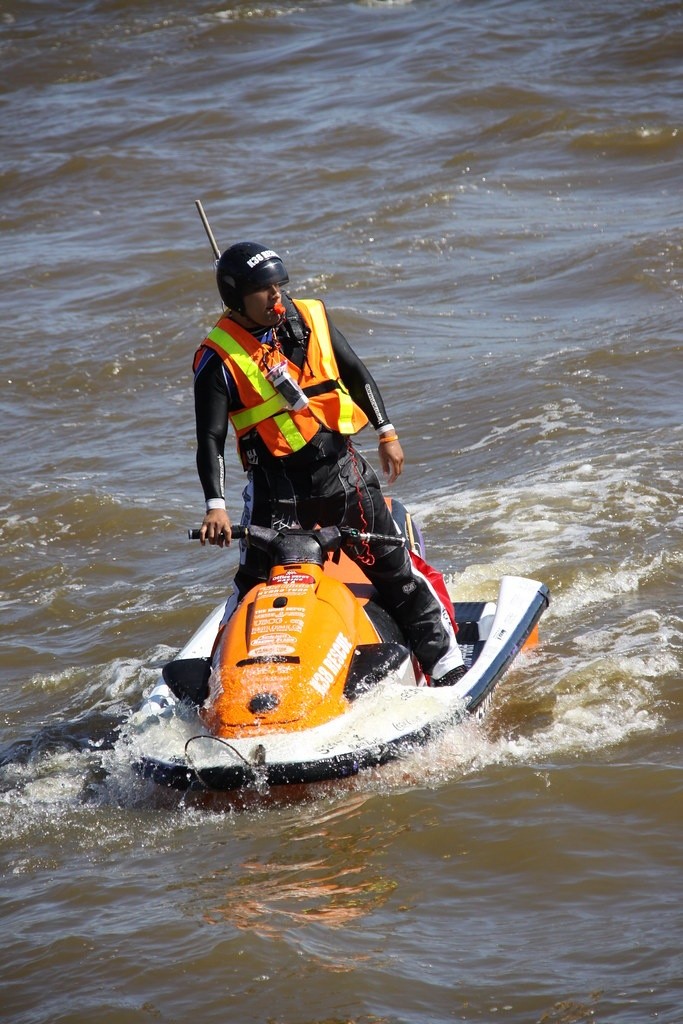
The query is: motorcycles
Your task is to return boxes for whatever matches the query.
[128,498,552,790]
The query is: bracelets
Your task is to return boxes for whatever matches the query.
[380,435,398,442]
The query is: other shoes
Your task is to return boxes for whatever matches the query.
[435,666,467,687]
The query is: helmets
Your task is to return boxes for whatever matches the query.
[216,242,289,315]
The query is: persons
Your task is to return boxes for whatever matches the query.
[192,242,467,687]
